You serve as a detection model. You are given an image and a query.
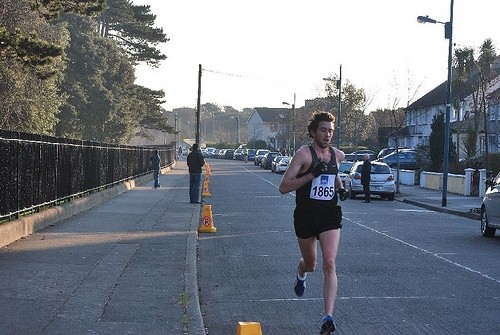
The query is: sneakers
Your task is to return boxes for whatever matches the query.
[294,270,307,297]
[320,314,337,335]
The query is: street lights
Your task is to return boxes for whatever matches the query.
[417,1,454,207]
[282,94,296,157]
[322,64,343,150]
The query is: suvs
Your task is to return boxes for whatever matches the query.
[336,146,417,201]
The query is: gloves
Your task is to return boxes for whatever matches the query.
[339,188,349,201]
[312,159,328,178]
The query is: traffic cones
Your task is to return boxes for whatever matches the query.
[199,205,216,232]
[201,160,212,196]
[237,322,262,335]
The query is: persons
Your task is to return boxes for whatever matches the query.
[186,144,205,204]
[178,147,183,157]
[242,144,248,163]
[360,153,371,203]
[152,150,161,189]
[278,111,348,335]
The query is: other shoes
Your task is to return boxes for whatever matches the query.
[363,201,369,203]
[195,201,201,204]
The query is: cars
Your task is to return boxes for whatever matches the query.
[480,168,500,236]
[182,144,293,174]
